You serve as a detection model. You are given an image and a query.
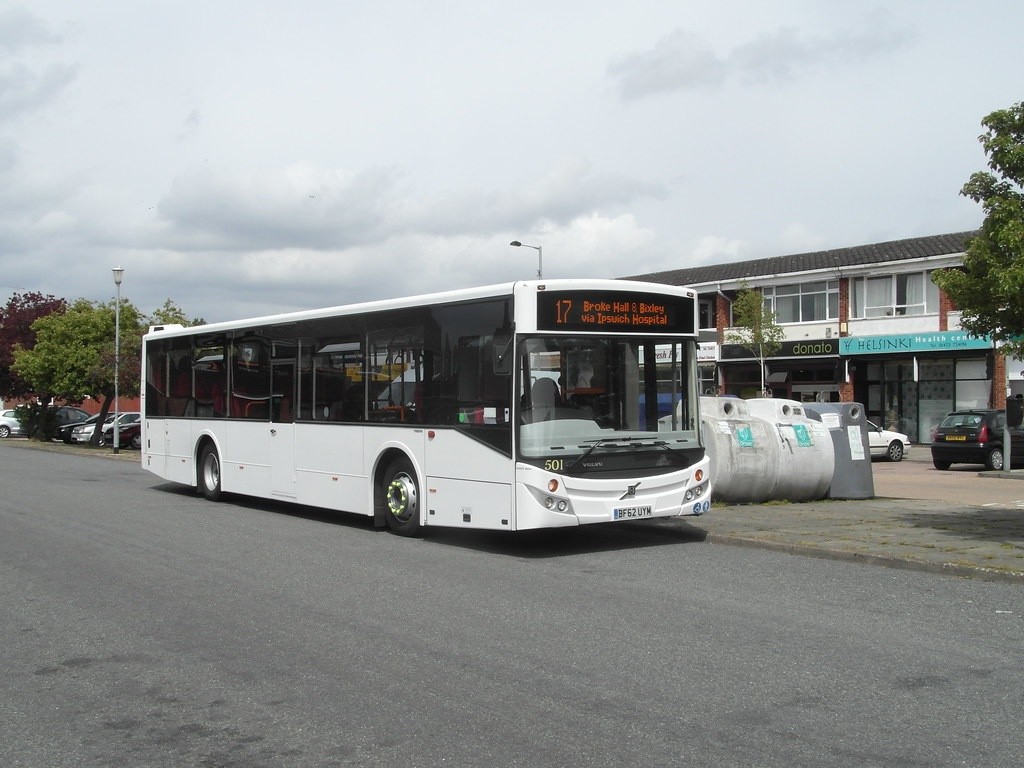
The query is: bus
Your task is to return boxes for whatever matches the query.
[139,278,713,539]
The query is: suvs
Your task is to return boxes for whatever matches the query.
[931,408,1023,471]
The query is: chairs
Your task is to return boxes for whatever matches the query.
[532,378,562,409]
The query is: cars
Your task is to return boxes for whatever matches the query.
[103,417,141,451]
[39,405,93,438]
[56,411,115,446]
[866,420,912,462]
[71,411,141,444]
[0,407,29,439]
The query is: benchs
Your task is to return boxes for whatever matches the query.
[156,362,458,422]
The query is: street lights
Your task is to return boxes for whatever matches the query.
[511,240,544,280]
[110,266,126,453]
